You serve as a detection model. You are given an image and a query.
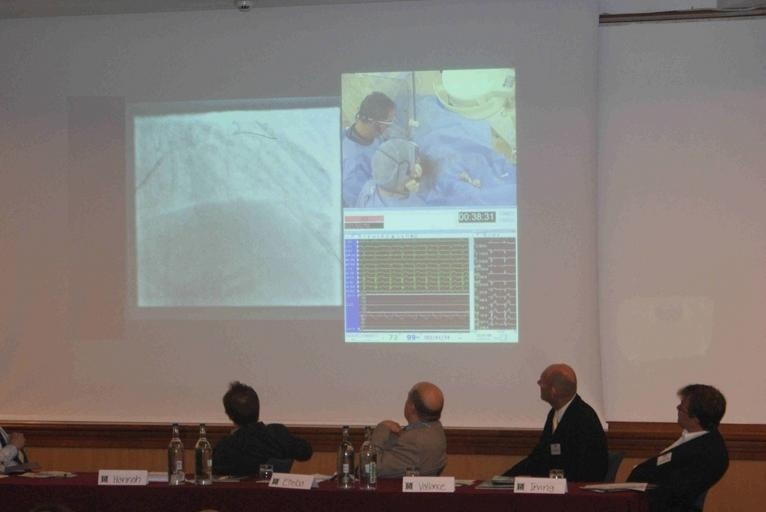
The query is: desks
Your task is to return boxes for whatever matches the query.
[0,471,656,512]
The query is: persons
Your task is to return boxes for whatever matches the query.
[0,426,33,475]
[354,139,433,207]
[490,363,608,482]
[341,91,400,207]
[622,382,728,511]
[345,380,449,478]
[202,378,313,476]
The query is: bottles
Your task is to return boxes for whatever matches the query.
[195,423,214,486]
[359,425,377,492]
[166,422,186,486]
[336,425,356,491]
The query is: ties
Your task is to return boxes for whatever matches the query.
[552,416,559,433]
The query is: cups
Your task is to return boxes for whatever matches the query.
[549,469,565,479]
[405,470,419,476]
[258,464,273,481]
